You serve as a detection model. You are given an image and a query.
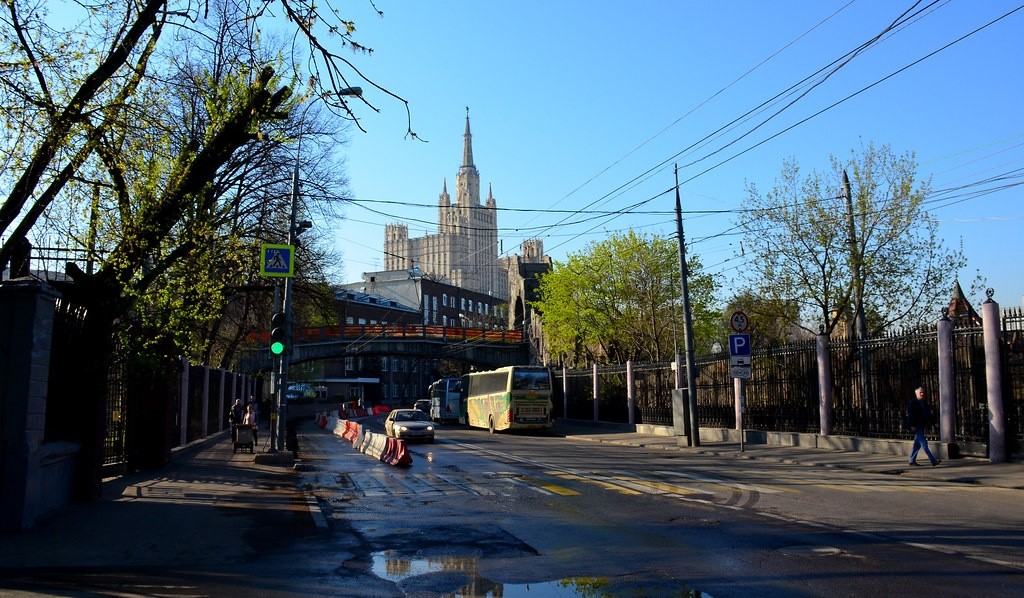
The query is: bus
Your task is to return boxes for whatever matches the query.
[428,379,462,424]
[456,366,555,434]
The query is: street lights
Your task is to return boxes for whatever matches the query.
[268,87,364,461]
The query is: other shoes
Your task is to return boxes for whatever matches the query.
[910,461,920,466]
[933,459,941,466]
[255,442,257,446]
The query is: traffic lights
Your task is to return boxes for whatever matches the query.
[269,313,286,358]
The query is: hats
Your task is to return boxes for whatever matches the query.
[235,399,240,403]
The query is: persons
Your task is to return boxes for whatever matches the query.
[358,397,362,409]
[241,394,260,423]
[909,386,942,467]
[413,414,419,419]
[243,404,259,446]
[228,398,245,442]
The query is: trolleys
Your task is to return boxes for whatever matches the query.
[233,424,255,454]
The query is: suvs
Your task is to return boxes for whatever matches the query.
[414,399,430,415]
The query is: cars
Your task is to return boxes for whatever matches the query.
[384,408,435,444]
